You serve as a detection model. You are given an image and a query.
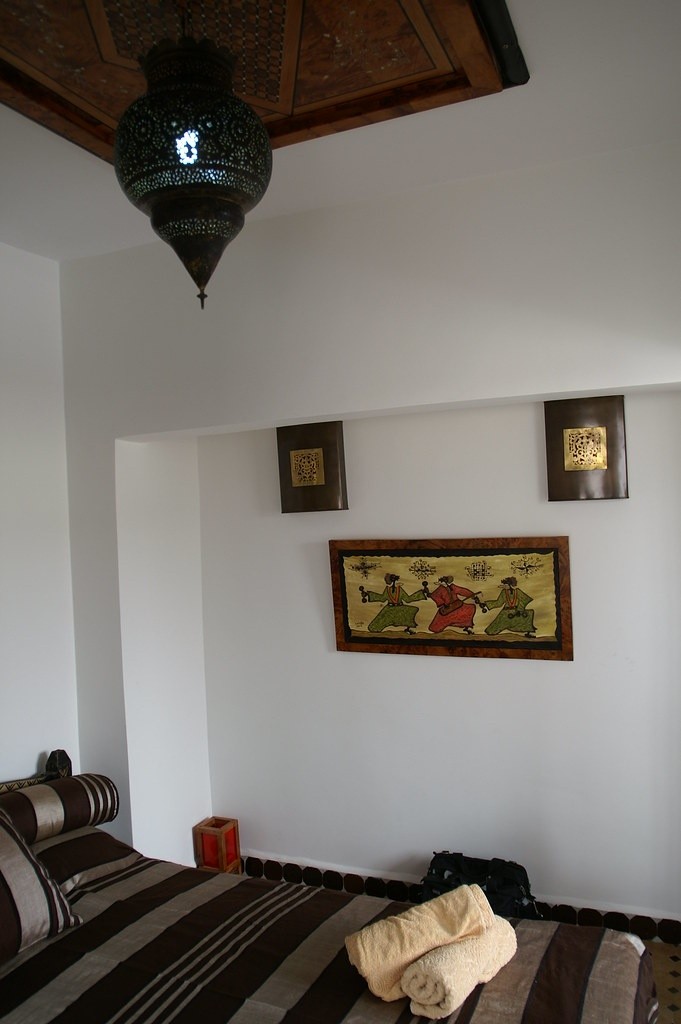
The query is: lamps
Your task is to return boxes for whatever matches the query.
[114,9,274,309]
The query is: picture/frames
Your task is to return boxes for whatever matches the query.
[327,535,577,665]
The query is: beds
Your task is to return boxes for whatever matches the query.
[0,745,681,1024]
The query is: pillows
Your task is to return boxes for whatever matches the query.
[28,827,149,904]
[0,772,119,844]
[0,807,85,978]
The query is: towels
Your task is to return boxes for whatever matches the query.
[401,913,518,1021]
[344,883,496,1001]
